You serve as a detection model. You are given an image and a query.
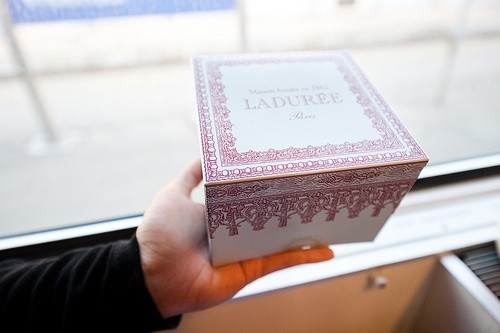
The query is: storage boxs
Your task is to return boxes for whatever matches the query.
[192,50,430,268]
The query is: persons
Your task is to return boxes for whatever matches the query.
[0,156,334,332]
